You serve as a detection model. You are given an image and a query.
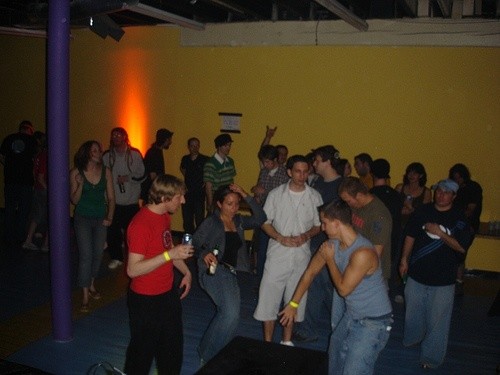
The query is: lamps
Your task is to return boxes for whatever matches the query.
[88,12,126,42]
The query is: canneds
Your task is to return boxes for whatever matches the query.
[182,233,192,245]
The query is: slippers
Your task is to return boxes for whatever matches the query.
[89,286,101,300]
[79,303,89,312]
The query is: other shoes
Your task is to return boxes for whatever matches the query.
[108,259,123,269]
[41,246,49,251]
[22,241,39,250]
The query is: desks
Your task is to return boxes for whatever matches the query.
[193,334,330,375]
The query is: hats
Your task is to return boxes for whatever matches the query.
[371,158,391,179]
[215,133,233,148]
[431,178,459,193]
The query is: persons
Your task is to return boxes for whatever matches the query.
[179,137,211,236]
[399,179,471,369]
[252,154,324,348]
[192,185,268,366]
[203,133,237,217]
[277,198,394,375]
[70,140,115,312]
[250,123,483,341]
[140,128,174,205]
[0,120,48,253]
[122,173,195,375]
[102,127,148,270]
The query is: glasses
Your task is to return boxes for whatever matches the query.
[112,134,123,138]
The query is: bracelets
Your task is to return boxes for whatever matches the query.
[288,301,299,308]
[164,251,171,262]
